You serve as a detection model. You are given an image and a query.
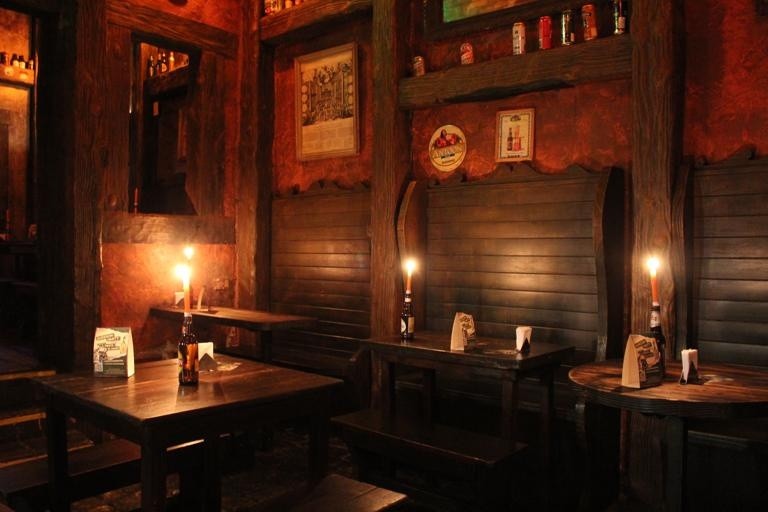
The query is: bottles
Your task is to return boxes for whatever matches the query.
[513,125,521,151]
[647,304,665,371]
[177,312,199,386]
[145,48,175,77]
[399,292,415,340]
[506,127,514,151]
[1,49,34,70]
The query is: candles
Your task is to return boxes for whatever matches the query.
[184,277,190,314]
[650,270,659,305]
[406,267,411,292]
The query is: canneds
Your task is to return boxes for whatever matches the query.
[537,16,554,51]
[560,9,575,46]
[611,0,627,36]
[411,56,425,80]
[459,43,474,66]
[581,5,598,41]
[512,23,526,55]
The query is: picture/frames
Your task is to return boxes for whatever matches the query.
[293,41,363,163]
[494,107,537,163]
